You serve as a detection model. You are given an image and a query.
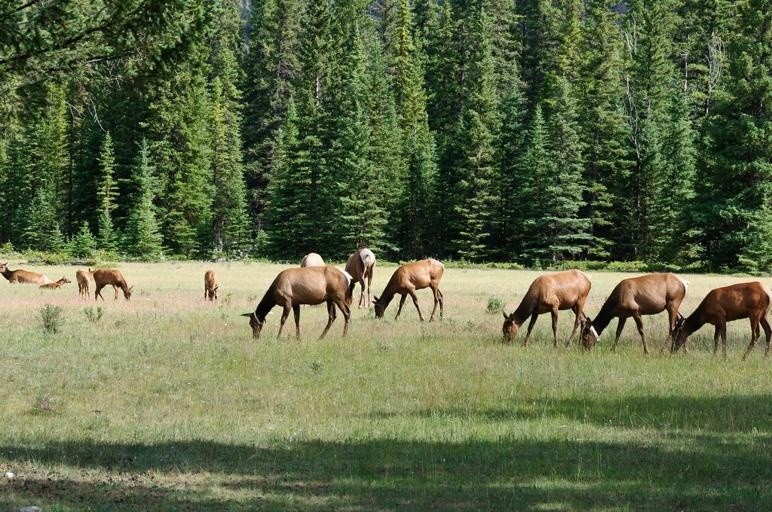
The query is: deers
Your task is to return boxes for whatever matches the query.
[204,269,219,302]
[76,268,97,299]
[371,257,447,322]
[668,280,772,362]
[0,262,46,284]
[38,274,71,289]
[579,272,686,355]
[89,268,134,301]
[500,269,592,348]
[239,265,351,339]
[344,247,376,310]
[300,252,326,266]
